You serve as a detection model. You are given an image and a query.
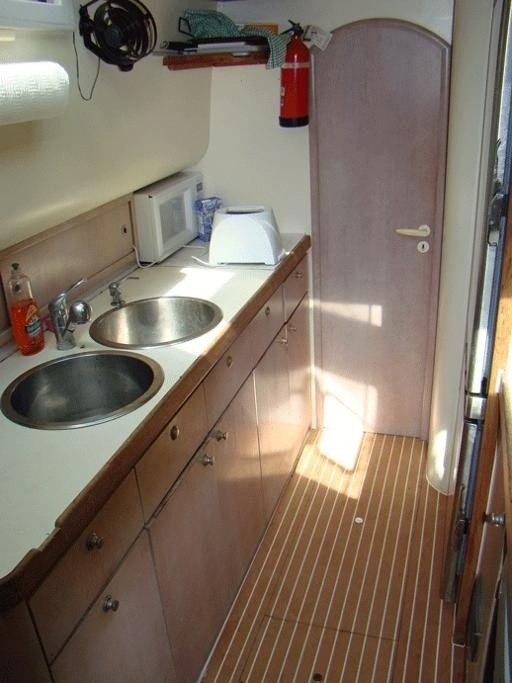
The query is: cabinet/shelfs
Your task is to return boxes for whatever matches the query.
[462,388,512,683]
[250,255,312,540]
[125,319,268,679]
[0,451,182,682]
[0,0,77,33]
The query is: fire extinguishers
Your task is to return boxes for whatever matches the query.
[279,19,310,127]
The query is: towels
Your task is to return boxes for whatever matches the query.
[0,61,70,125]
[183,8,287,70]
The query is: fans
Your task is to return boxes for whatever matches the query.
[79,0,157,71]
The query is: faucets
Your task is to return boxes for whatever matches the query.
[108,276,140,306]
[48,275,92,350]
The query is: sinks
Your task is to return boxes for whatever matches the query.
[0,349,166,430]
[89,295,225,350]
